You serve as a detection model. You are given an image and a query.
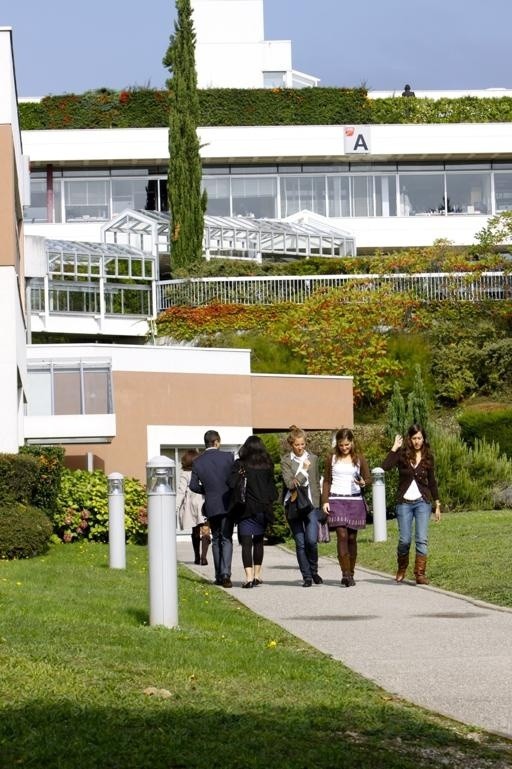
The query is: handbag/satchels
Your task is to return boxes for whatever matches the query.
[222,487,243,514]
[318,520,330,543]
[285,479,314,522]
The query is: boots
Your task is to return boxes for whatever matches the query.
[414,554,429,584]
[191,534,200,564]
[396,552,409,582]
[338,553,352,587]
[350,552,356,586]
[201,534,210,565]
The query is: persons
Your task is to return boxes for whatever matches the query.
[234,435,279,587]
[190,431,234,586]
[280,425,323,587]
[382,425,442,583]
[175,448,210,564]
[321,428,372,587]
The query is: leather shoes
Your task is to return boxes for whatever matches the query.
[312,574,323,584]
[215,577,223,585]
[303,580,312,587]
[241,582,253,588]
[253,579,262,585]
[222,574,232,588]
